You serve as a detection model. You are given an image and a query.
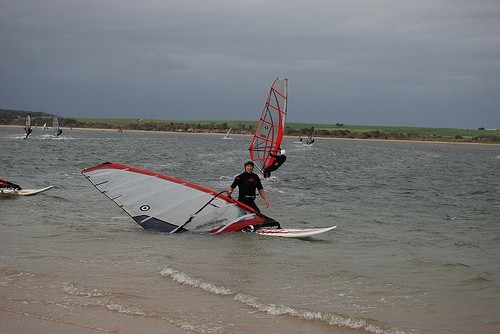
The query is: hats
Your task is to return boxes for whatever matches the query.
[245,159,254,170]
[280,149,286,155]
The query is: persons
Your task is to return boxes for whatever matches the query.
[227,160,269,214]
[57,129,62,136]
[24,127,33,139]
[263,145,287,177]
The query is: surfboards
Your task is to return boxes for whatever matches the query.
[255,225,337,238]
[0,185,55,196]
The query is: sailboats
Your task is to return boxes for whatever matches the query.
[247,78,289,183]
[52,114,60,137]
[23,114,33,139]
[0,179,55,196]
[297,132,302,143]
[220,128,233,141]
[81,161,337,239]
[305,129,314,144]
[41,123,48,131]
